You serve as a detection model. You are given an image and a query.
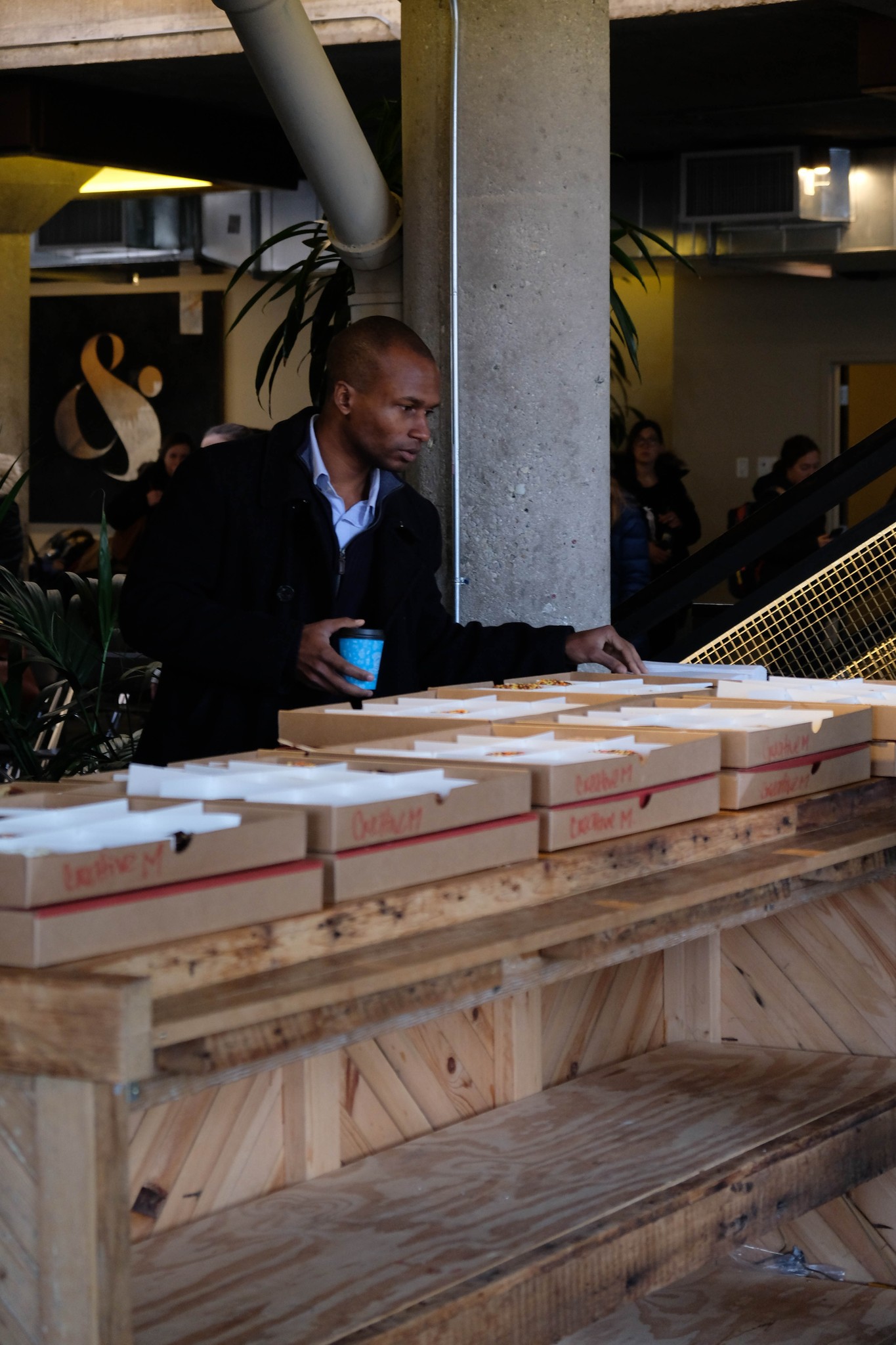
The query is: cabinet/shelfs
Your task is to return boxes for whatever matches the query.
[0,777,896,1343]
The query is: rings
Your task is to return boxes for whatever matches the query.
[670,516,673,520]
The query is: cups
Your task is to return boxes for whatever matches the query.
[339,626,385,691]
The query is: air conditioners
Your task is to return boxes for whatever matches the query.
[679,146,800,224]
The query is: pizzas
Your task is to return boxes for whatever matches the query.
[494,680,571,690]
[481,747,643,763]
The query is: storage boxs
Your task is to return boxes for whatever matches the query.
[0,659,896,970]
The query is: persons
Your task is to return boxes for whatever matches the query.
[726,433,835,679]
[607,452,651,664]
[610,418,702,664]
[0,422,273,591]
[118,314,645,769]
